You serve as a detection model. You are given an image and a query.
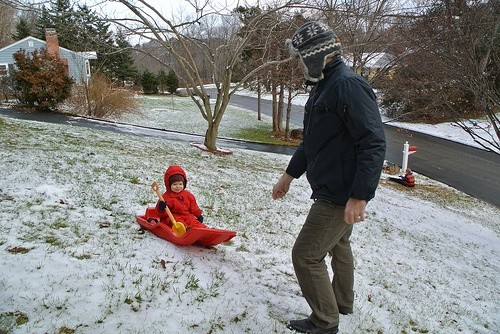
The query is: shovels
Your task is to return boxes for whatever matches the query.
[151,181,186,236]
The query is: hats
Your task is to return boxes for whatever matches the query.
[286,20,344,84]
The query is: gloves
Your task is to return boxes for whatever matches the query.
[157,200,168,210]
[197,215,204,223]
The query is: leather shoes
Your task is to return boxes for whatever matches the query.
[283,314,338,334]
[338,305,353,315]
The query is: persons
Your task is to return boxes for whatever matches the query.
[272,19,386,334]
[155,166,208,231]
